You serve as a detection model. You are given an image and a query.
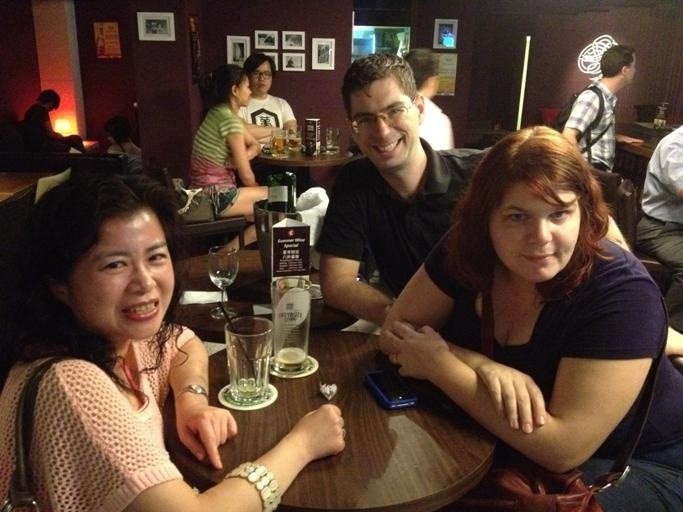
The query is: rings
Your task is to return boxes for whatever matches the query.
[393,354,398,364]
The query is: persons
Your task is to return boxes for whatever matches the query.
[561,44,637,173]
[635,125,683,336]
[189,63,270,253]
[103,115,142,172]
[0,157,346,512]
[312,51,492,331]
[378,124,683,512]
[236,52,298,149]
[151,22,164,34]
[402,47,454,152]
[21,88,71,152]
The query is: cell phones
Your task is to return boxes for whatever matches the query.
[366,370,420,410]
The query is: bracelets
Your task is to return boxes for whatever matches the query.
[224,461,281,512]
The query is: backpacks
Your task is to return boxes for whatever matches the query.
[550,85,605,148]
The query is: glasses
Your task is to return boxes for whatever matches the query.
[247,70,272,81]
[351,96,416,135]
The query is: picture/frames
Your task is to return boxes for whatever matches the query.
[259,52,279,75]
[279,30,306,50]
[281,51,305,72]
[430,17,459,50]
[251,29,279,51]
[134,9,177,43]
[223,33,251,70]
[310,36,337,72]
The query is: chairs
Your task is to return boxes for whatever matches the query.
[609,174,672,290]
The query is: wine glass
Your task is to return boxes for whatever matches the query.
[207,245,240,320]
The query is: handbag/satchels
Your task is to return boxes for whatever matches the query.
[448,443,629,512]
[171,187,215,223]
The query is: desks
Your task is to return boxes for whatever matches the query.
[162,135,496,510]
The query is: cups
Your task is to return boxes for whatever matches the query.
[270,276,312,374]
[266,125,340,159]
[225,317,276,406]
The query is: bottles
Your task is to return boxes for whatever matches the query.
[652,102,670,130]
[266,172,296,217]
